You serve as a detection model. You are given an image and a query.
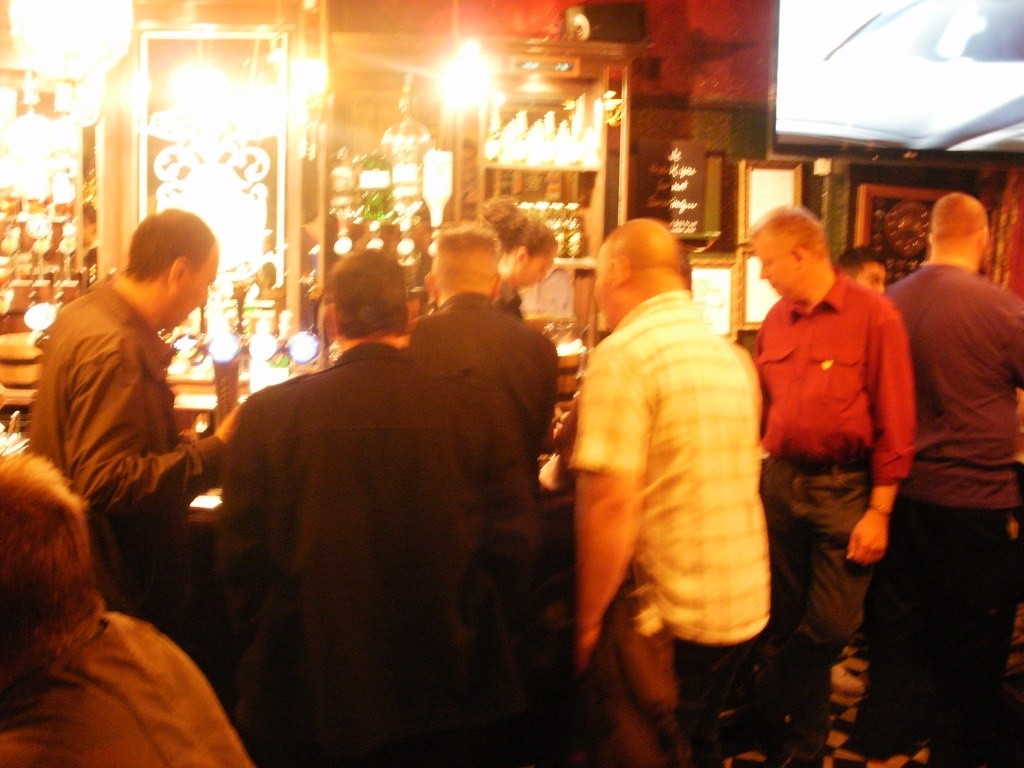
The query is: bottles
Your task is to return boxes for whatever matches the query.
[0,108,583,289]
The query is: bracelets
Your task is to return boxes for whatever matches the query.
[868,503,892,516]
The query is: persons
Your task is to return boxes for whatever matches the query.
[838,246,887,295]
[408,225,560,467]
[854,194,1024,768]
[0,452,253,768]
[214,248,540,768]
[478,196,558,320]
[24,209,242,632]
[565,216,772,768]
[748,204,918,768]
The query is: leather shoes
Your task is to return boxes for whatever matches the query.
[747,661,784,755]
[758,747,822,768]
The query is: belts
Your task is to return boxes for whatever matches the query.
[776,454,868,475]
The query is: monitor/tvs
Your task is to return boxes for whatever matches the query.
[766,0,1024,166]
